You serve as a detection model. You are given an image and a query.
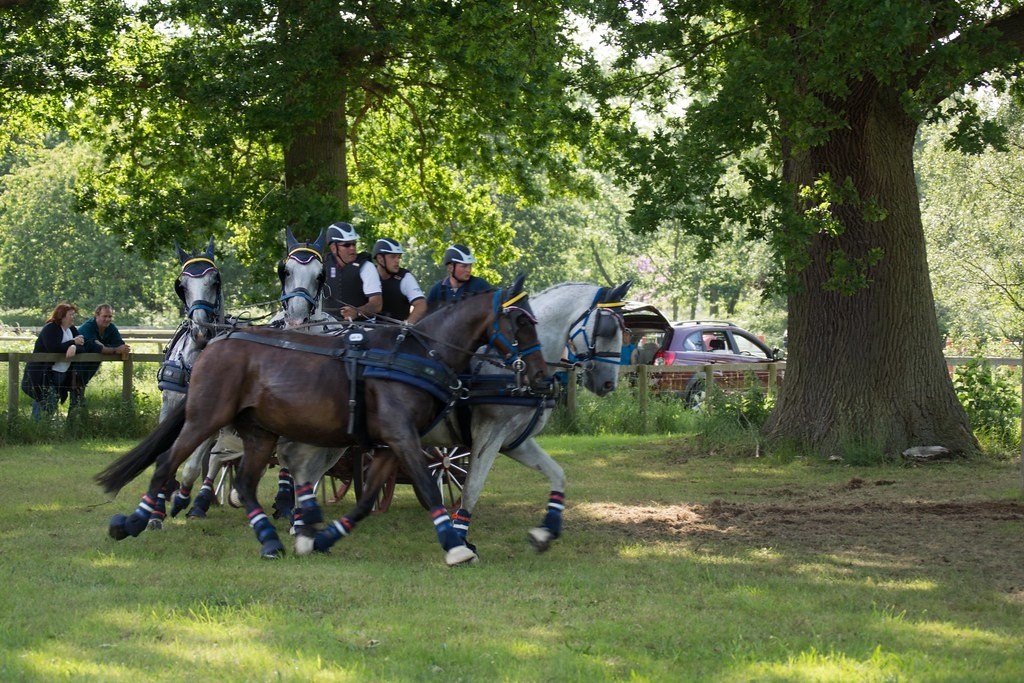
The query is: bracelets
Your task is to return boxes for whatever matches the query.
[113,347,116,354]
[357,312,362,317]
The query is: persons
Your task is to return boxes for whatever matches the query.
[321,221,382,319]
[427,244,490,310]
[20,304,84,418]
[68,304,130,414]
[621,332,639,366]
[372,238,427,324]
[703,334,716,351]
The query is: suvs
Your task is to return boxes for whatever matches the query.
[615,301,789,412]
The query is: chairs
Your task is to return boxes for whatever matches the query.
[641,343,658,361]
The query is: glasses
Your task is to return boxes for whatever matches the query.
[335,242,357,248]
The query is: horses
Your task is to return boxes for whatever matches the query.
[147,235,247,533]
[266,226,344,521]
[108,271,553,570]
[278,278,636,555]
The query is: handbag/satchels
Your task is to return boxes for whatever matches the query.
[66,369,76,392]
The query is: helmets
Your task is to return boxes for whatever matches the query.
[371,237,406,259]
[326,222,361,246]
[445,245,475,263]
